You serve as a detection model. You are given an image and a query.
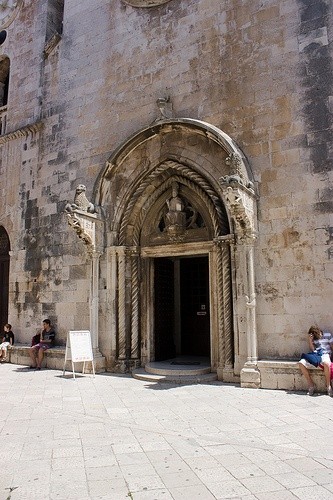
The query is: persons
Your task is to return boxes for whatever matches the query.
[297,326,333,398]
[0,324,15,362]
[164,182,186,237]
[27,319,57,370]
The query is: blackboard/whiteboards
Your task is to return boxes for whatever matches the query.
[65,330,93,362]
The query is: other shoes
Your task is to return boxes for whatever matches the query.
[2,355,8,361]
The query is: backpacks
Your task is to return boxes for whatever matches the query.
[31,334,41,346]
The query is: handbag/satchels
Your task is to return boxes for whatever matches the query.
[301,349,324,368]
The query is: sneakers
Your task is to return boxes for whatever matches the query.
[327,386,333,397]
[309,383,317,396]
[29,365,37,369]
[33,367,41,370]
